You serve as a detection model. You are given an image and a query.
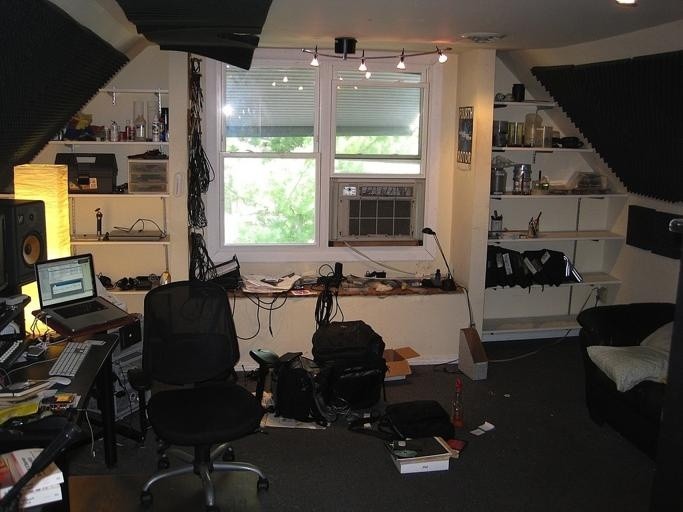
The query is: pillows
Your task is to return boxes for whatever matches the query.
[583,312,677,394]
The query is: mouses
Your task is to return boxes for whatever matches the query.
[48,376,71,385]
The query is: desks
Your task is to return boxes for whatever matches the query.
[0,330,122,512]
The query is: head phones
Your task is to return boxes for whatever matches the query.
[116,276,138,291]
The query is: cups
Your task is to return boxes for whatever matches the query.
[529,223,539,238]
[493,112,553,148]
[490,220,502,238]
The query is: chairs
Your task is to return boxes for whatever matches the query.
[132,278,270,510]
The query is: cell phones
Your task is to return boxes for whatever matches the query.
[27,348,45,359]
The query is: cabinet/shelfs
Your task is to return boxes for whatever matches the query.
[480,100,626,344]
[40,86,172,300]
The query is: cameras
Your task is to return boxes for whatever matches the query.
[149,273,161,283]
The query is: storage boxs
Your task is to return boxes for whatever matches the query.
[382,342,420,380]
[127,157,169,196]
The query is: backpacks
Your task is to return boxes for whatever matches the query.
[271,352,338,427]
[312,319,387,410]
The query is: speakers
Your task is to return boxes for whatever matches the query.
[1,198,47,287]
[107,319,144,361]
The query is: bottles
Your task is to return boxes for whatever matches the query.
[491,163,532,195]
[107,107,169,142]
[434,269,442,286]
[451,375,466,427]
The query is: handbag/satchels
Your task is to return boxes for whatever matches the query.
[384,399,455,442]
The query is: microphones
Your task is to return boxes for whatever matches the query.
[31,421,83,473]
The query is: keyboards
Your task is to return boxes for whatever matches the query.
[277,352,303,362]
[49,340,92,377]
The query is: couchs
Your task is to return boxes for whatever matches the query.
[576,298,678,463]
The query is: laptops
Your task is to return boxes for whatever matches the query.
[33,253,128,334]
[108,230,162,240]
